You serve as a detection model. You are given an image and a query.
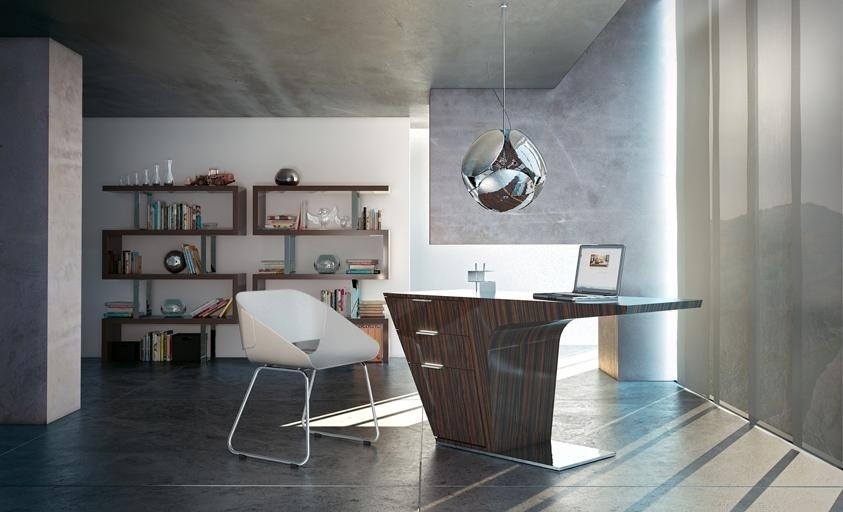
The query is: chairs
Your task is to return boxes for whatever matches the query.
[222,287,383,471]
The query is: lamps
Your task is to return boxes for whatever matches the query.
[456,0,551,219]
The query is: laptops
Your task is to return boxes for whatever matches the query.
[533,244,626,302]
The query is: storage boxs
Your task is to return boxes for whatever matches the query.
[170,330,215,366]
[104,340,140,363]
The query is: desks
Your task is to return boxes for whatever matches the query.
[379,290,705,475]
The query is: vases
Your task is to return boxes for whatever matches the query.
[117,156,175,188]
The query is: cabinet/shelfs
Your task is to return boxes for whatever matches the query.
[100,184,392,368]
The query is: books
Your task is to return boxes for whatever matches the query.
[258,206,388,318]
[145,194,218,231]
[117,250,142,274]
[180,243,199,275]
[103,297,233,362]
